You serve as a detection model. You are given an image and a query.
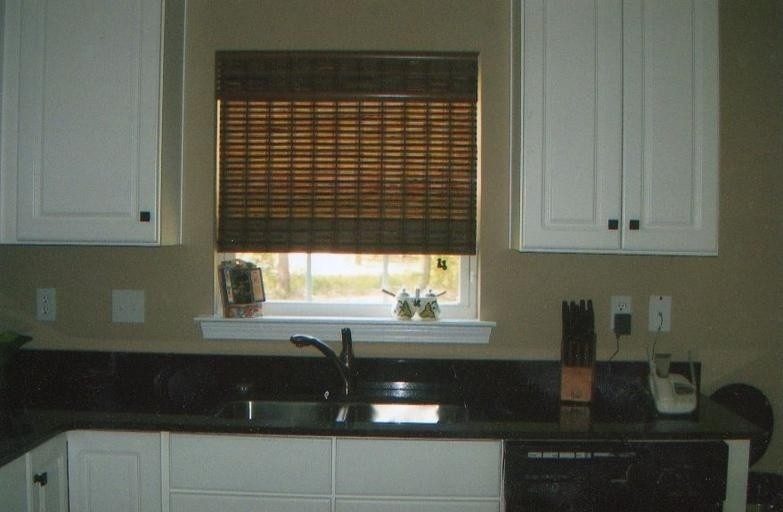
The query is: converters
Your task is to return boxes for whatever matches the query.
[616,311,633,339]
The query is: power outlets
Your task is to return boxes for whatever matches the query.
[111,288,145,324]
[35,288,56,321]
[649,295,673,333]
[612,295,637,333]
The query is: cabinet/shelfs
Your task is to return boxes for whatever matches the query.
[69,431,167,511]
[161,433,502,511]
[0,433,68,511]
[506,1,718,257]
[1,1,166,248]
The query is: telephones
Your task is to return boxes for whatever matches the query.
[649,350,698,415]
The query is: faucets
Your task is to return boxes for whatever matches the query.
[289,327,362,396]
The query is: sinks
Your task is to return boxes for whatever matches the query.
[214,398,340,428]
[339,400,476,432]
